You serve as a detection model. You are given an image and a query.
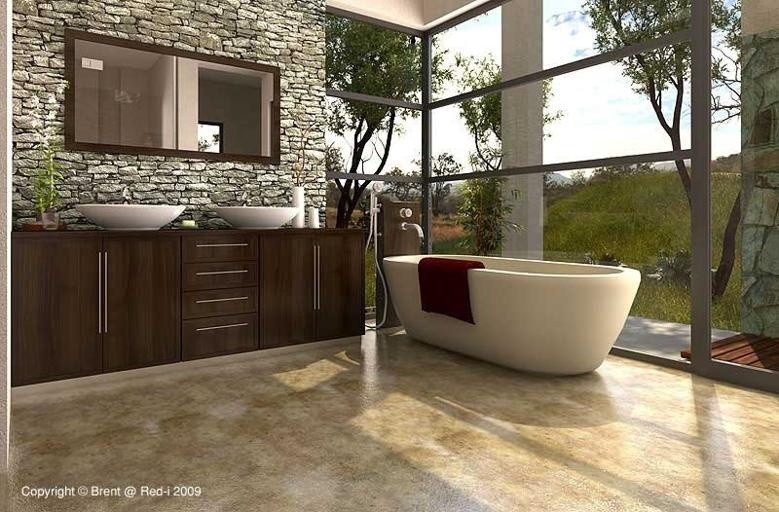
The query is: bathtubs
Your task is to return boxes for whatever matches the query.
[384,253,642,376]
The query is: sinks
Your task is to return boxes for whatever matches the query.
[75,204,185,231]
[212,206,299,229]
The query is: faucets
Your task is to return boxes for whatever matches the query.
[402,222,423,239]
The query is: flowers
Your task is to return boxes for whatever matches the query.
[25,79,76,211]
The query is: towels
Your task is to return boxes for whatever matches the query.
[417,256,487,326]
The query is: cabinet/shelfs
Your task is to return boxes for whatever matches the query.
[177,227,259,365]
[252,224,370,351]
[8,227,180,396]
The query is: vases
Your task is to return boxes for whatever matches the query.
[290,184,306,229]
[308,207,320,229]
[40,204,60,231]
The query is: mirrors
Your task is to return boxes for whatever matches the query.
[63,24,282,166]
